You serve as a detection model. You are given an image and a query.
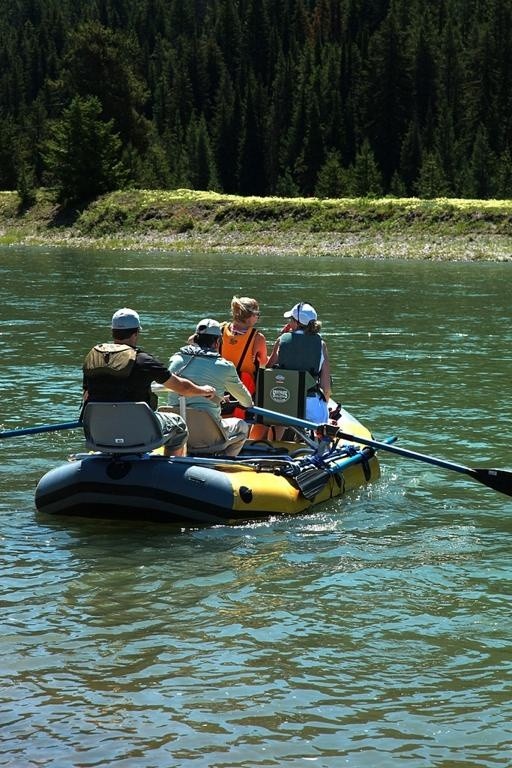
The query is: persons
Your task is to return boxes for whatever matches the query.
[167,316,253,457]
[264,300,332,442]
[216,296,267,442]
[82,307,216,458]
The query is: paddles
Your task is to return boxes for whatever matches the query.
[225,399,512,497]
[295,433,400,497]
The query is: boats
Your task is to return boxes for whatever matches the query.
[33,396,382,523]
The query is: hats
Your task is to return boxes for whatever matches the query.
[195,318,224,336]
[111,307,143,330]
[283,302,318,325]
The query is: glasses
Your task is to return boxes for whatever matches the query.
[296,301,312,325]
